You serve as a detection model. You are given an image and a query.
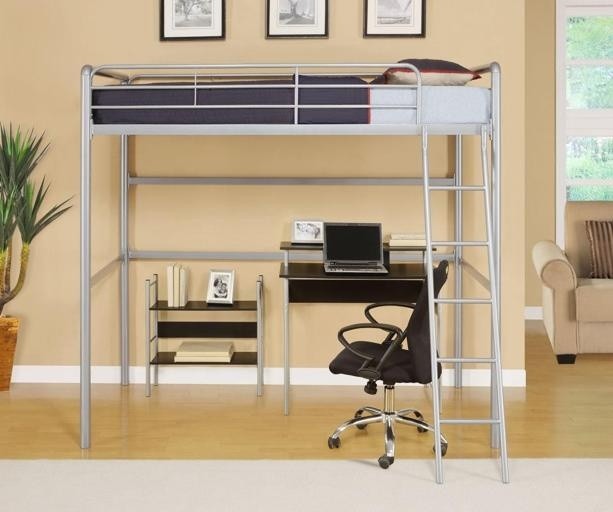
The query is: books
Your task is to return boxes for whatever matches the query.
[389,231,427,265]
[173,352,234,363]
[179,266,188,307]
[166,262,175,308]
[176,341,232,356]
[173,263,181,308]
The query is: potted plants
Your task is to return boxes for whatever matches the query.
[0,121,77,392]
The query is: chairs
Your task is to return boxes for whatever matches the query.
[326,259,449,468]
[530,240,613,364]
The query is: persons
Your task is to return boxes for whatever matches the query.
[212,280,227,298]
[215,283,226,298]
[296,222,320,239]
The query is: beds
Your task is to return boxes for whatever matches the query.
[79,62,503,450]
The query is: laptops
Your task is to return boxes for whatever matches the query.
[323,223,390,274]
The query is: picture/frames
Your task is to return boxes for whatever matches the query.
[363,0,426,37]
[291,217,325,244]
[206,268,235,305]
[159,0,226,40]
[266,0,328,37]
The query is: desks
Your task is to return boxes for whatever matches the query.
[278,239,438,415]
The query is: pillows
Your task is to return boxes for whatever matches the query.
[584,220,612,279]
[369,58,482,85]
[562,200,613,277]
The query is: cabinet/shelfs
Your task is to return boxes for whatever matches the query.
[143,272,265,397]
